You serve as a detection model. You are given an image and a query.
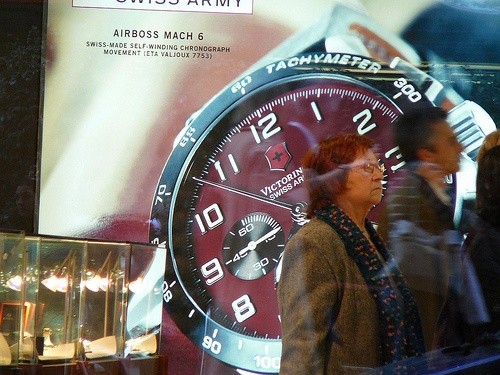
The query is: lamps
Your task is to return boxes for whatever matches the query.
[7,247,29,291]
[40,247,131,293]
[126,254,159,293]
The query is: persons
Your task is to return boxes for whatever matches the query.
[378,107,465,375]
[276,132,429,375]
[434,129,500,374]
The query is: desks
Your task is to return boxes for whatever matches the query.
[12,354,162,375]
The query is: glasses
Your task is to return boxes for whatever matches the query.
[351,159,384,174]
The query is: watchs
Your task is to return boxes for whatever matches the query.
[147,2,497,374]
